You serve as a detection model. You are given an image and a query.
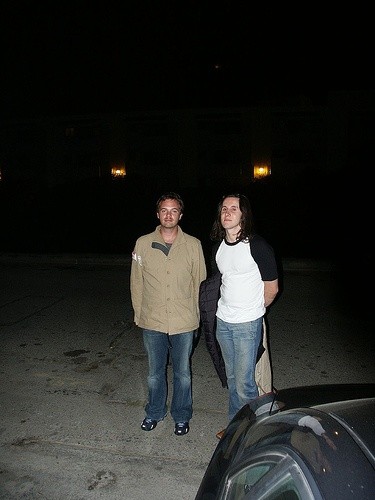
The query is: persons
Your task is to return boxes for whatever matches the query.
[206,192,279,441]
[129,192,208,435]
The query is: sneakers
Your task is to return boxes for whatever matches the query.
[174,422,189,436]
[140,417,157,431]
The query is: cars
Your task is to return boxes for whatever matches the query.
[194,382,375,499]
